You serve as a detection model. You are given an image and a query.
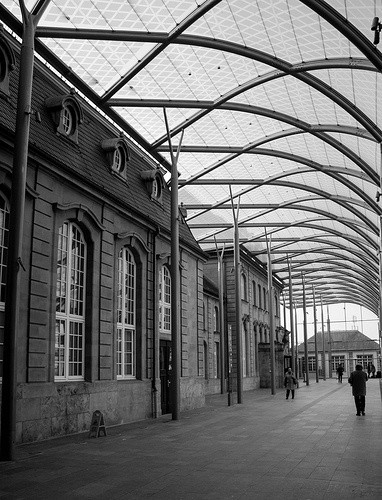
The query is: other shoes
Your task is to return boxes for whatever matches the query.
[362,413,365,416]
[356,414,360,416]
[286,397,288,399]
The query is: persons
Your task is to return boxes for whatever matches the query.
[336,363,344,383]
[284,367,298,399]
[348,364,375,416]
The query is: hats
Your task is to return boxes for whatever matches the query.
[356,365,363,369]
